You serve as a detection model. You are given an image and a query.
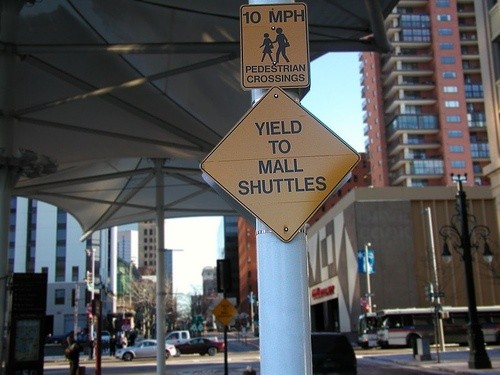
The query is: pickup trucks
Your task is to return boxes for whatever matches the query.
[165,331,218,346]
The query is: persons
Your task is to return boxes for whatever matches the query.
[108,332,117,357]
[119,333,128,350]
[240,322,249,345]
[63,332,81,374]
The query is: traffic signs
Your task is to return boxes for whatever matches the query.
[199,84,361,244]
[239,2,311,89]
[211,298,239,326]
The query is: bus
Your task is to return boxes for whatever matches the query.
[377,305,500,349]
[358,312,377,349]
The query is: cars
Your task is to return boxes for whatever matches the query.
[176,338,224,356]
[115,340,176,361]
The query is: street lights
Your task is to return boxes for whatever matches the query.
[439,173,494,368]
